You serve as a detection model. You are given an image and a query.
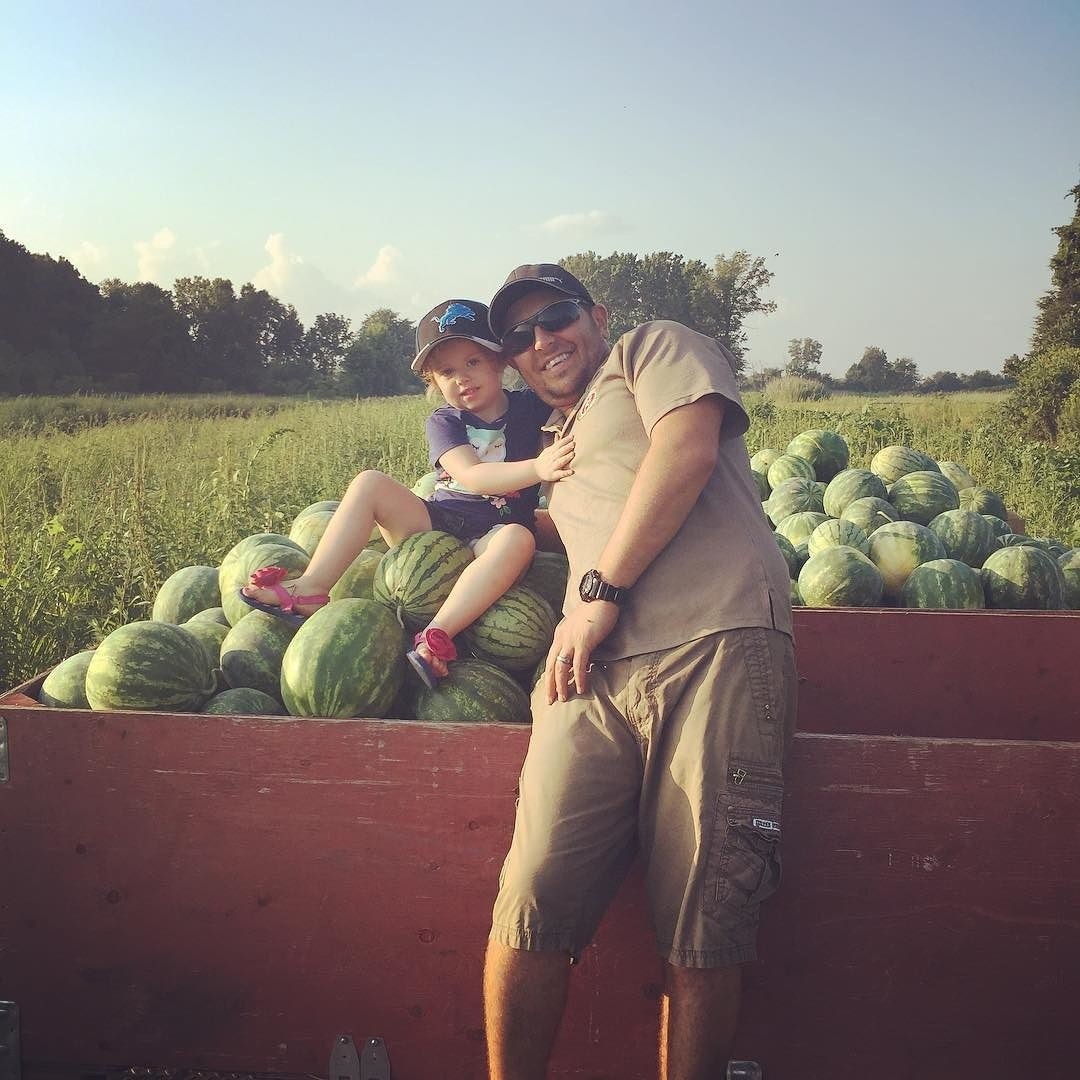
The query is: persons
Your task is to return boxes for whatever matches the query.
[485,262,798,1080]
[237,298,576,691]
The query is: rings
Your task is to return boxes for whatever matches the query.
[557,655,571,664]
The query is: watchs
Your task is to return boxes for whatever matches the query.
[579,568,629,604]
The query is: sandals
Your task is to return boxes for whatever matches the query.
[238,567,330,625]
[406,628,458,691]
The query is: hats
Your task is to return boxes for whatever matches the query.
[488,264,595,341]
[411,300,504,372]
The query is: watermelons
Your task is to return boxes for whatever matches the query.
[744,430,1080,611]
[40,472,572,722]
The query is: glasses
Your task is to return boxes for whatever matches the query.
[500,299,589,357]
